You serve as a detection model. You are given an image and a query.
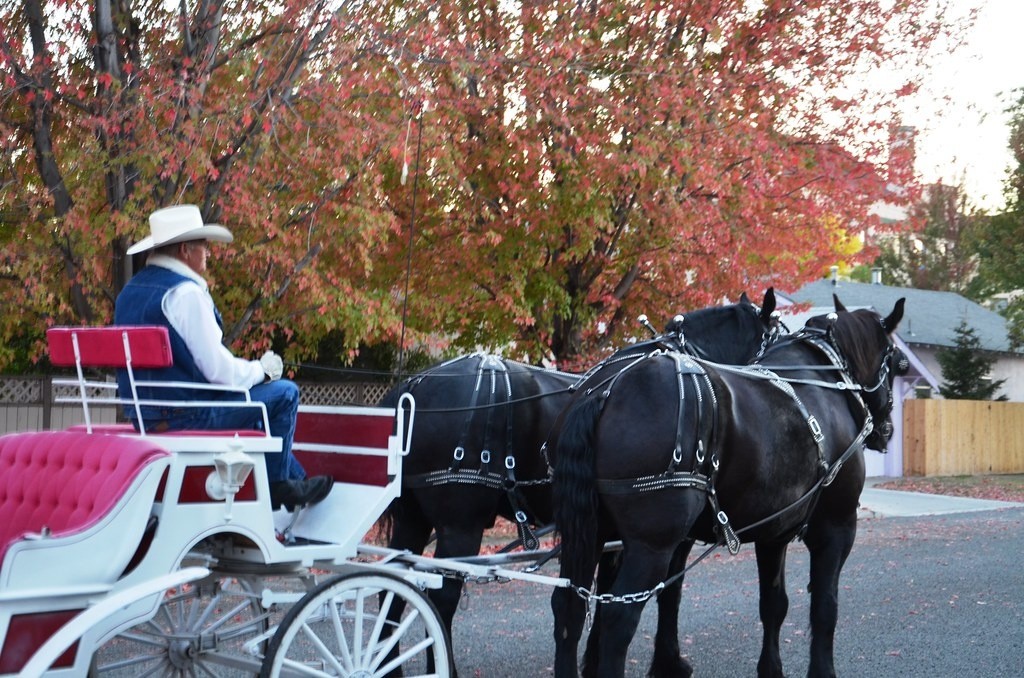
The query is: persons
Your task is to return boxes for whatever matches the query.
[113,202,336,515]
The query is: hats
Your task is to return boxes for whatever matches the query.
[126,204,233,255]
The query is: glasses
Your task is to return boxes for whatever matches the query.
[193,240,210,252]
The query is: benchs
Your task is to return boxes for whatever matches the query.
[0,433,173,600]
[47,326,332,548]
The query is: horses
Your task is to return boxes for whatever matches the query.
[373,284,906,678]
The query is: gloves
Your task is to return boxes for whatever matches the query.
[260,350,283,384]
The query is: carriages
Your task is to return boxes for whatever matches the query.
[0,286,905,678]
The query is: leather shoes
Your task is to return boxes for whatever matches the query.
[269,474,334,512]
